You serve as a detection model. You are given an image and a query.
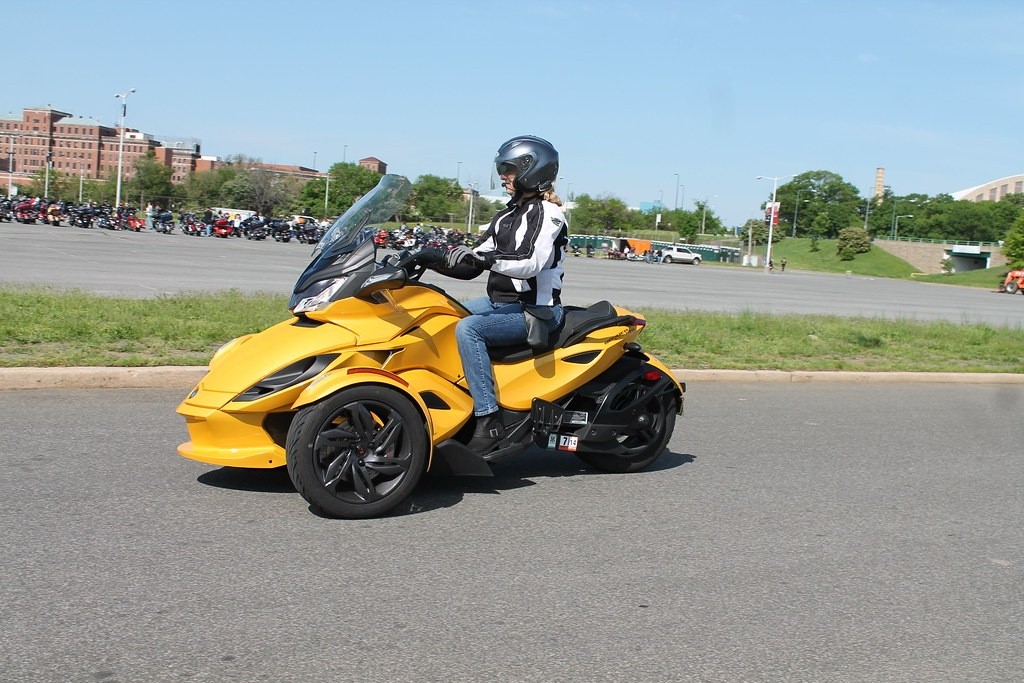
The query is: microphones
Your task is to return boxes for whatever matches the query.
[502,182,506,187]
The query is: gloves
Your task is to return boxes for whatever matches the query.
[448,245,486,268]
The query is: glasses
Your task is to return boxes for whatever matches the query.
[497,163,516,175]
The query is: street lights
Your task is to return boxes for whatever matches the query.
[114,88,136,212]
[792,188,818,237]
[674,173,680,212]
[456,161,463,182]
[559,176,573,205]
[701,193,719,234]
[889,198,916,241]
[468,182,478,233]
[893,215,915,240]
[313,151,318,169]
[864,185,891,233]
[680,184,686,209]
[325,172,331,215]
[343,144,348,161]
[756,174,799,270]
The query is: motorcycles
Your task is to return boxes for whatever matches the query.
[173,173,687,520]
[0,194,658,258]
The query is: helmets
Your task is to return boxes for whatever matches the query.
[490,134,559,207]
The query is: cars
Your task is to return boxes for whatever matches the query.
[661,246,703,265]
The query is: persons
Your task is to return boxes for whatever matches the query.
[145,201,161,230]
[400,221,407,229]
[84,198,128,226]
[413,223,423,235]
[218,210,241,225]
[623,244,635,257]
[394,135,568,459]
[648,245,662,265]
[781,258,787,272]
[202,207,213,236]
[15,192,41,205]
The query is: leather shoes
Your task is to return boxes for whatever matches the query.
[467,414,509,457]
